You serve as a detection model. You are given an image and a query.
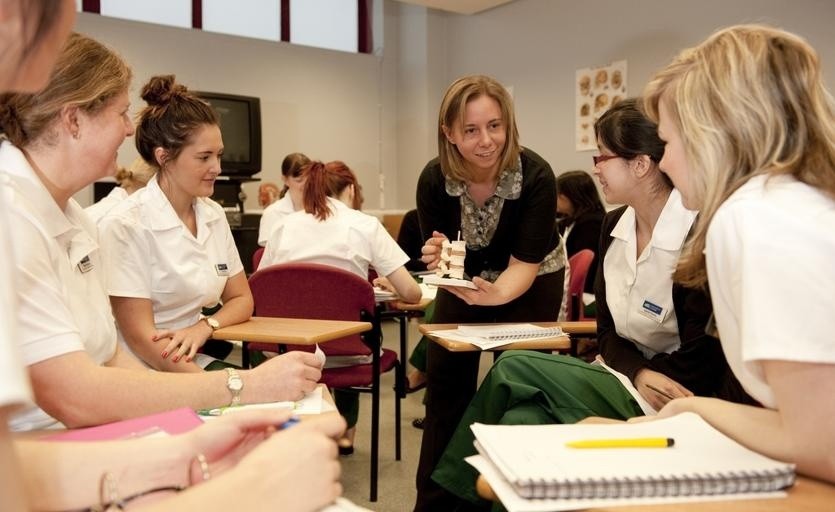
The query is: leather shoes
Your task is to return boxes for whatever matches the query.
[392,375,428,394]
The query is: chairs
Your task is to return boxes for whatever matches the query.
[240,261,402,503]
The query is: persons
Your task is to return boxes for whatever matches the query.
[431,95,763,512]
[642,22,835,511]
[257,150,312,247]
[82,155,160,223]
[98,71,255,374]
[0,29,323,428]
[257,160,423,305]
[0,0,346,512]
[555,169,607,316]
[414,72,565,512]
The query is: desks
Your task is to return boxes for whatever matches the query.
[475,415,835,512]
[417,319,598,352]
[210,316,373,356]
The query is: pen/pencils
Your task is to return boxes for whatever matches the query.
[197,400,301,417]
[567,437,673,450]
[281,419,351,449]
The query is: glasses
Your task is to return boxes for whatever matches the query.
[592,154,621,166]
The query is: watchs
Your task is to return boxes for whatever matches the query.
[200,317,221,331]
[224,367,244,407]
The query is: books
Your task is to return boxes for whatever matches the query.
[469,411,798,499]
[455,322,564,343]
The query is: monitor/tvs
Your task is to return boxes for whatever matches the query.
[190,91,261,177]
